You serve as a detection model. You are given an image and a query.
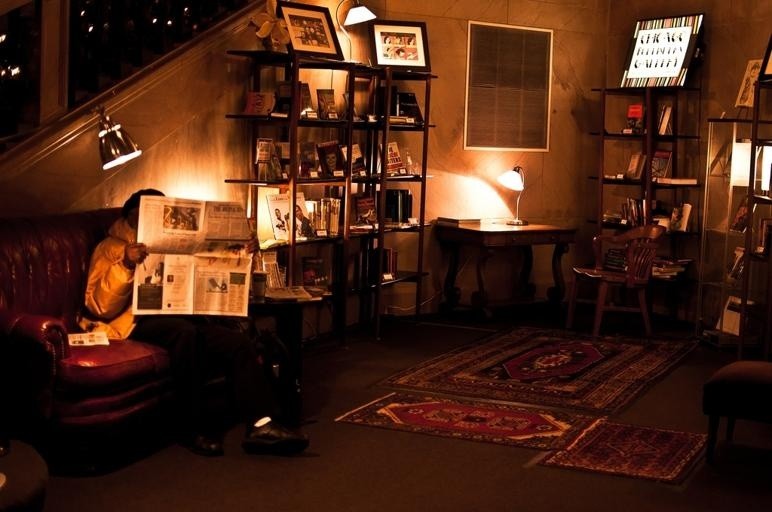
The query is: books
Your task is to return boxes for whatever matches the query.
[603,102,694,280]
[246,82,426,283]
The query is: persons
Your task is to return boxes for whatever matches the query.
[83,187,310,457]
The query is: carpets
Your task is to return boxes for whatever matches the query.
[334,327,707,489]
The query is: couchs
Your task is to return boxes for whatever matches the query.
[0,207,174,463]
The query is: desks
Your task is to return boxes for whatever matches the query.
[440,223,578,309]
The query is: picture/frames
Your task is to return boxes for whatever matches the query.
[369,21,432,72]
[276,1,345,60]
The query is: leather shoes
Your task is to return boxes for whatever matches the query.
[188,434,224,456]
[245,423,309,450]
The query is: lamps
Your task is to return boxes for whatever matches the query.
[498,166,528,225]
[96,104,142,170]
[334,0,377,65]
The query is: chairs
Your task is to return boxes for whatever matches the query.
[565,224,666,333]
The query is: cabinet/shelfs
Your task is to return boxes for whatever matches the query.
[587,87,701,237]
[223,49,436,344]
[695,79,771,361]
